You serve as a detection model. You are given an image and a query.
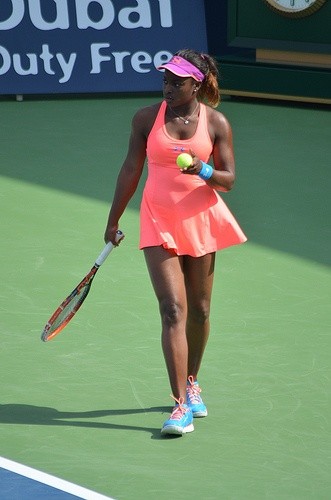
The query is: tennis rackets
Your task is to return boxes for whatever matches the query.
[42,229,125,342]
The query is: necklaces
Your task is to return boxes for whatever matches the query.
[167,102,199,125]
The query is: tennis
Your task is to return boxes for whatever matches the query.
[176,152,194,170]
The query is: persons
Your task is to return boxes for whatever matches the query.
[104,48,248,434]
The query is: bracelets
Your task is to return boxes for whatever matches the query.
[198,160,213,181]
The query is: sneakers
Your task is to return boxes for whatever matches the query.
[160,404,193,434]
[186,381,207,417]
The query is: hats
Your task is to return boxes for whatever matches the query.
[156,56,204,82]
